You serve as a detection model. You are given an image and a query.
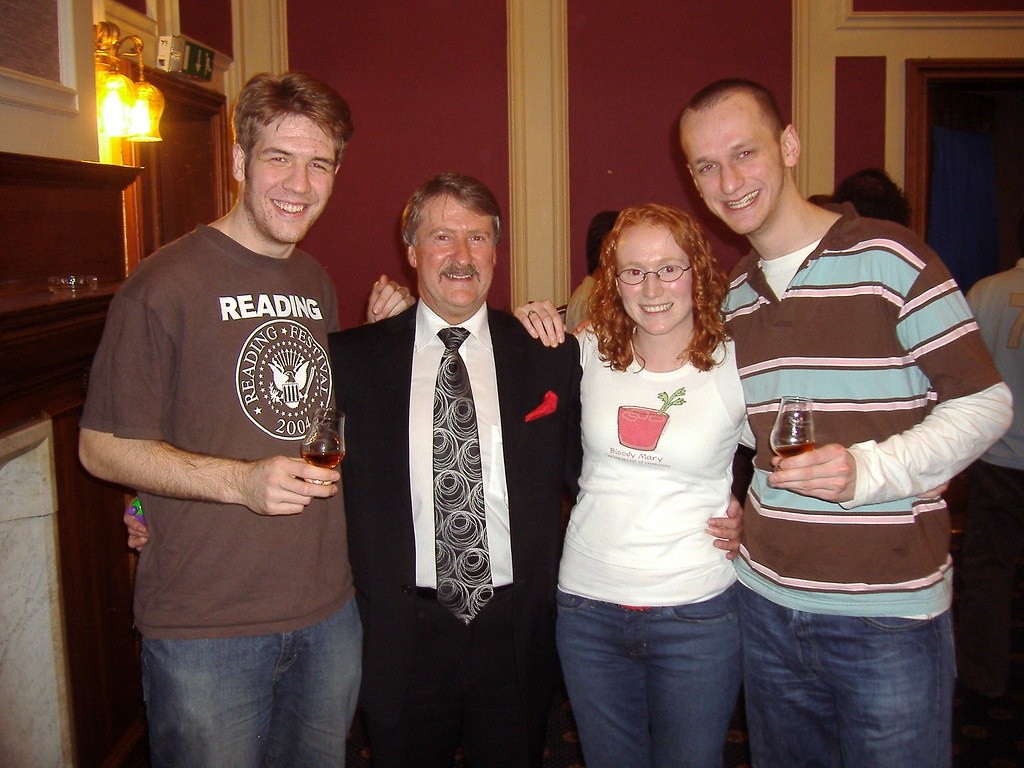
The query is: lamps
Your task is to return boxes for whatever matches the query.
[96,18,165,144]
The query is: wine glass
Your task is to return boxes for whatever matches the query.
[771,393,819,475]
[303,406,345,487]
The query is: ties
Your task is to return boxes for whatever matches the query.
[432,328,495,625]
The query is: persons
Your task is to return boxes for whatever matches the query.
[807,166,913,233]
[119,170,584,768]
[77,73,419,768]
[675,75,1017,768]
[553,211,626,326]
[514,200,748,765]
[958,254,1023,712]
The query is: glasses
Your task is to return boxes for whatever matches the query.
[611,265,694,286]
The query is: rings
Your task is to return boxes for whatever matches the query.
[525,306,538,321]
